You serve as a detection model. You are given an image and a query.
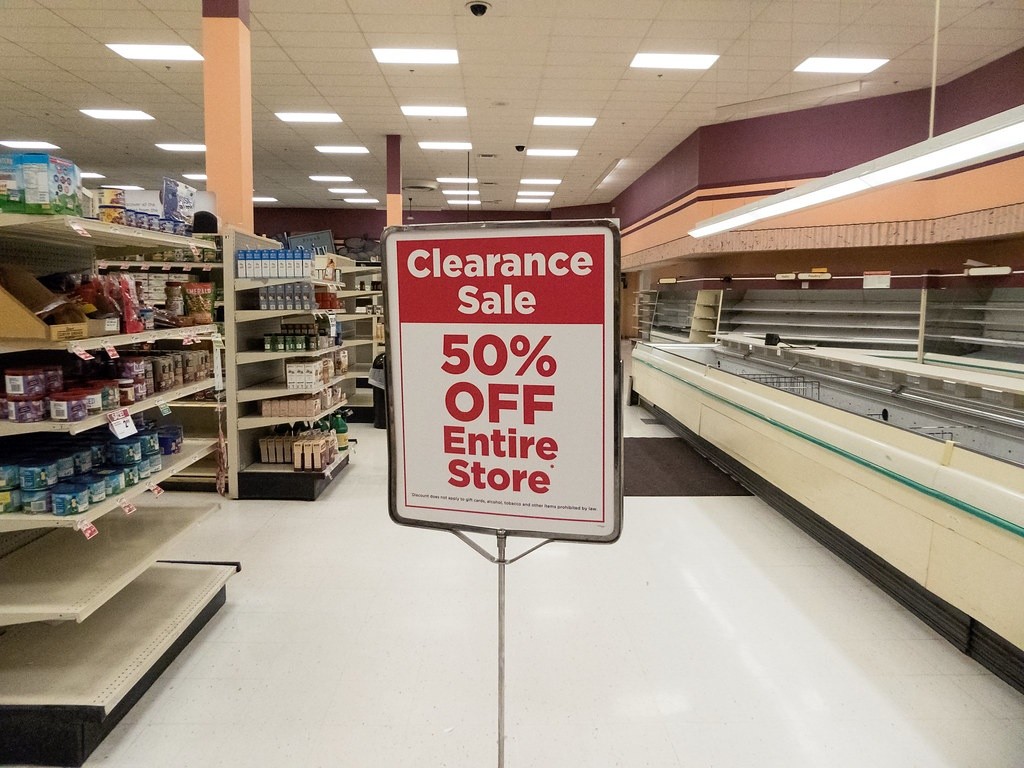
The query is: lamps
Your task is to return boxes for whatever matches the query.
[687,1,1024,239]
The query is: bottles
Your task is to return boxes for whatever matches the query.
[135,281,143,301]
[277,414,349,451]
[165,281,185,316]
[263,323,334,352]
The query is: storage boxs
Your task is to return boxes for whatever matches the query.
[239,249,348,472]
[0,152,83,217]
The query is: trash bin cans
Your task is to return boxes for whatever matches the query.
[373,352,386,429]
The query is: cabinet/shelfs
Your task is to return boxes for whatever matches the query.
[1,215,241,767]
[316,253,384,423]
[159,226,357,501]
[629,288,1024,396]
